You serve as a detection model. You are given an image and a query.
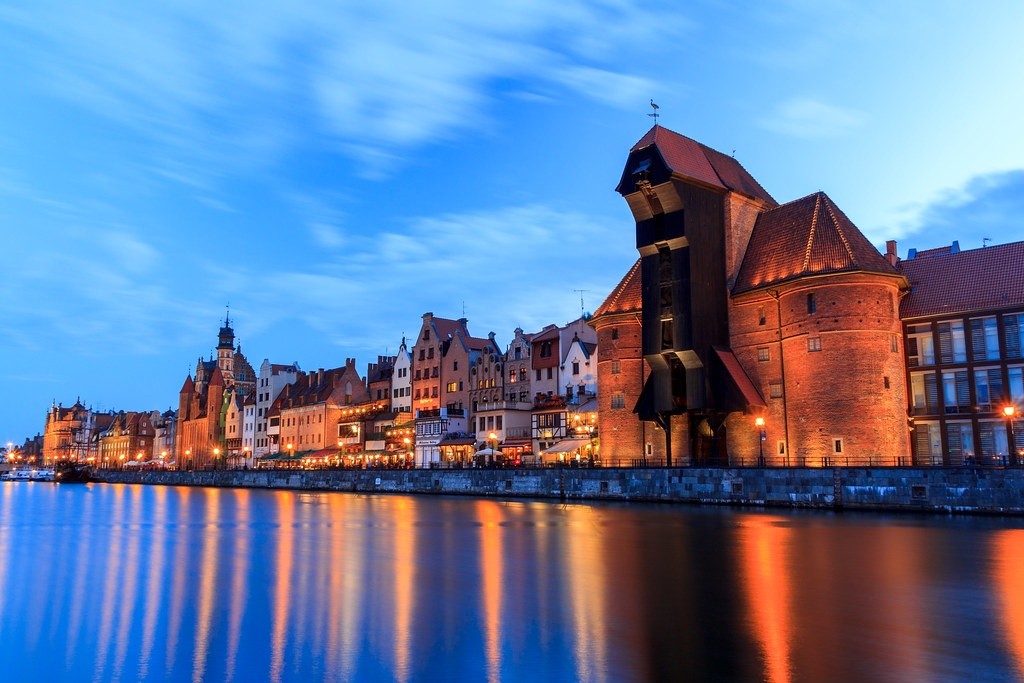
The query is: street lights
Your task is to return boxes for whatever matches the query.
[286,443,292,470]
[405,438,411,468]
[337,440,344,471]
[1004,404,1019,467]
[243,447,250,470]
[756,417,765,467]
[489,432,497,469]
[213,449,219,472]
[185,450,191,472]
[162,452,167,470]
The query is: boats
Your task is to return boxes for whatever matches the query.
[0,464,56,482]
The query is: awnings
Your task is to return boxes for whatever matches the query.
[262,452,285,460]
[543,440,590,453]
[282,451,315,461]
[303,449,340,458]
[227,453,246,458]
[436,438,476,446]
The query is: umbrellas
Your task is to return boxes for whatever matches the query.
[475,448,503,456]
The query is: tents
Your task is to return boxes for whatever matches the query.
[123,461,139,470]
[169,461,175,466]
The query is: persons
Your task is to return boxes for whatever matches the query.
[523,457,526,464]
[258,457,407,469]
